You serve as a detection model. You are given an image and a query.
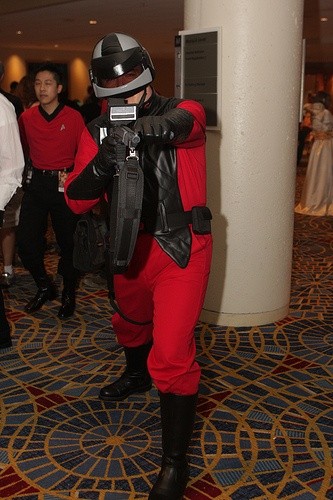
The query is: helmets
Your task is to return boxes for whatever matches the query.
[89,32,156,99]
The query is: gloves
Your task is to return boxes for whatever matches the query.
[100,126,137,167]
[135,117,174,147]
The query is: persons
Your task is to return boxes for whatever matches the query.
[64,32,214,500]
[0,61,103,347]
[295,91,333,216]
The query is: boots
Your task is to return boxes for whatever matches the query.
[98,346,152,400]
[147,392,198,500]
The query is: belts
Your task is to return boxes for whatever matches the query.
[31,169,74,177]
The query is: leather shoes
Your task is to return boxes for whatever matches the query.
[23,286,54,313]
[57,290,75,319]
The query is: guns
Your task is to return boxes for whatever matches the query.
[107,125,140,174]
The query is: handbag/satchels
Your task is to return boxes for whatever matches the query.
[70,218,109,273]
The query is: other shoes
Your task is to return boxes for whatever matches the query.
[0,271,15,287]
